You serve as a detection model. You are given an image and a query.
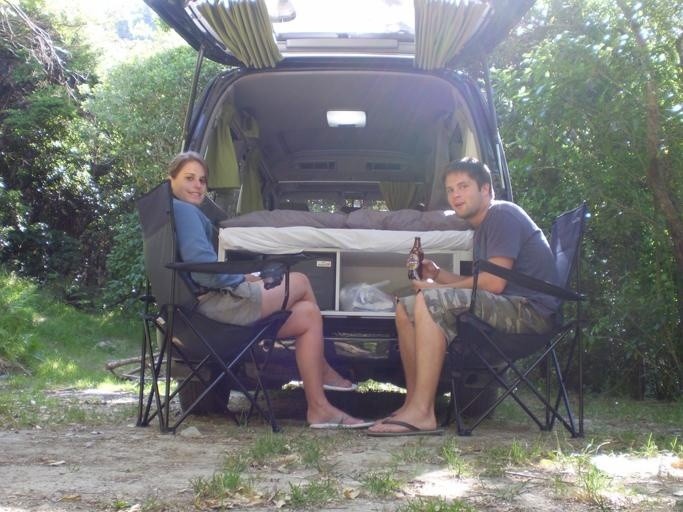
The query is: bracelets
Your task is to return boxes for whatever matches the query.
[432,266,440,281]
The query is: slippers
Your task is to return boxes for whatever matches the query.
[323,373,358,392]
[309,410,375,429]
[367,417,445,435]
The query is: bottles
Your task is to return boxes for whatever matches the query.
[407,236,423,281]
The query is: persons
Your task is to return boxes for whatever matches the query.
[368,156,566,434]
[167,150,374,429]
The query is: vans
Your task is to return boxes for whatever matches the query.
[141,0,545,420]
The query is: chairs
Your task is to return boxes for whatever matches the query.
[443,201,589,439]
[126,176,307,435]
